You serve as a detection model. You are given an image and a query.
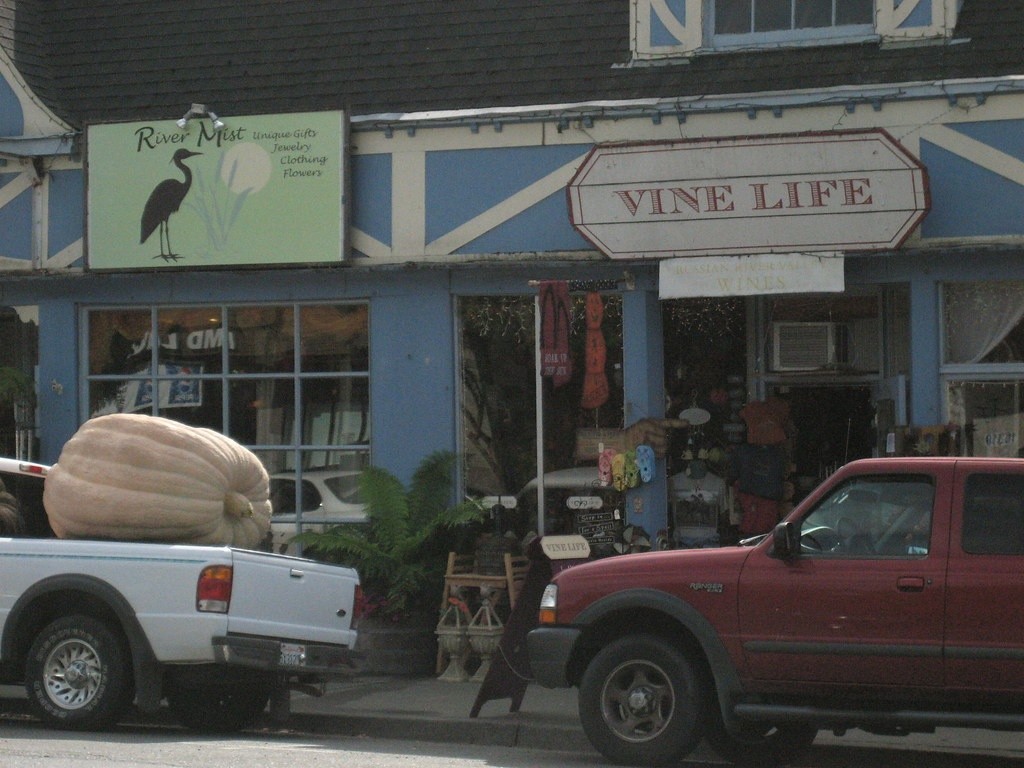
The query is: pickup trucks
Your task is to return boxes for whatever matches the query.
[0,459,361,731]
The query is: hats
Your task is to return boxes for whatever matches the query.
[521,531,539,555]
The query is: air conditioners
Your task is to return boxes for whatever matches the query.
[770,319,848,373]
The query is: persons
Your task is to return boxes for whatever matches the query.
[575,418,691,461]
[255,686,299,734]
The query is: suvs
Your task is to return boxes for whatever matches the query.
[526,453,1024,768]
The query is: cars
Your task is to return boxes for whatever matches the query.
[267,465,402,563]
[520,467,738,546]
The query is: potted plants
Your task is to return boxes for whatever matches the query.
[285,448,487,679]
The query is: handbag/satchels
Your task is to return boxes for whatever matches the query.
[714,401,796,548]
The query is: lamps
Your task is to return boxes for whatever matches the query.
[623,270,637,291]
[176,103,226,133]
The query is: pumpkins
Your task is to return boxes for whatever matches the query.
[43,413,273,549]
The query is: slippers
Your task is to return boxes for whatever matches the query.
[612,453,626,491]
[636,445,656,483]
[623,450,641,488]
[598,448,618,486]
